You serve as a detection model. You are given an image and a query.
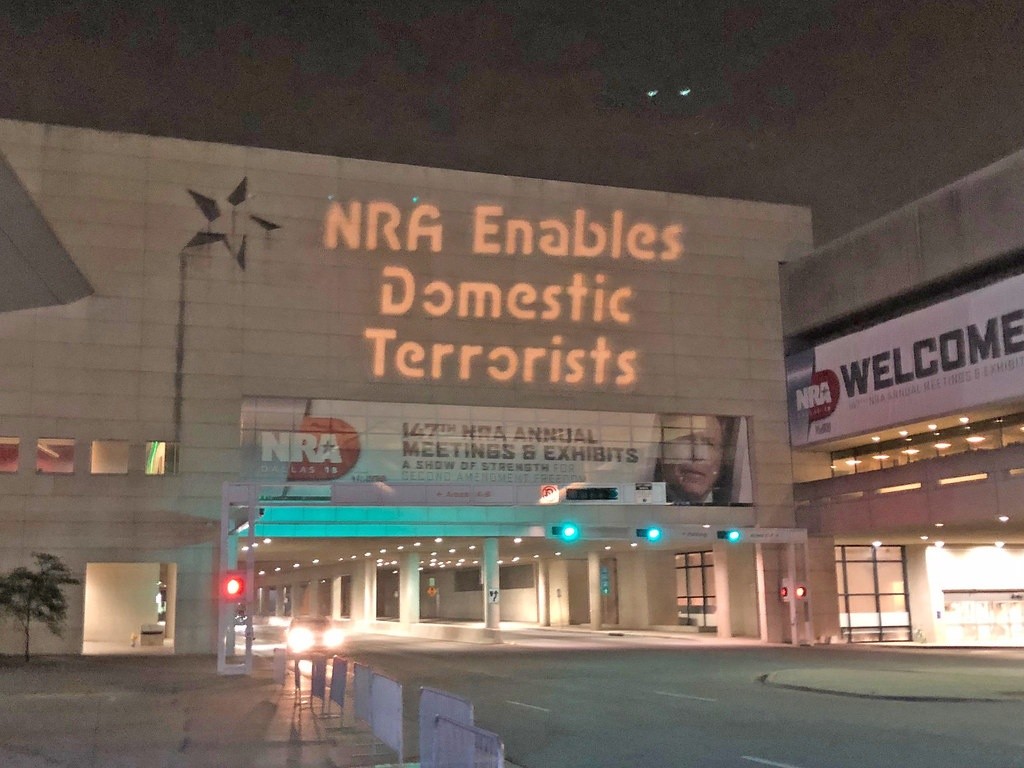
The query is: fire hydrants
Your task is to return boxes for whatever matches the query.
[130,634,137,647]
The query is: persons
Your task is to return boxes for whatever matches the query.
[649,413,728,505]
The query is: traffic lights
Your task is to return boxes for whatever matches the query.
[717,530,740,542]
[781,587,787,597]
[552,526,577,538]
[636,527,662,538]
[796,587,807,598]
[222,575,245,598]
